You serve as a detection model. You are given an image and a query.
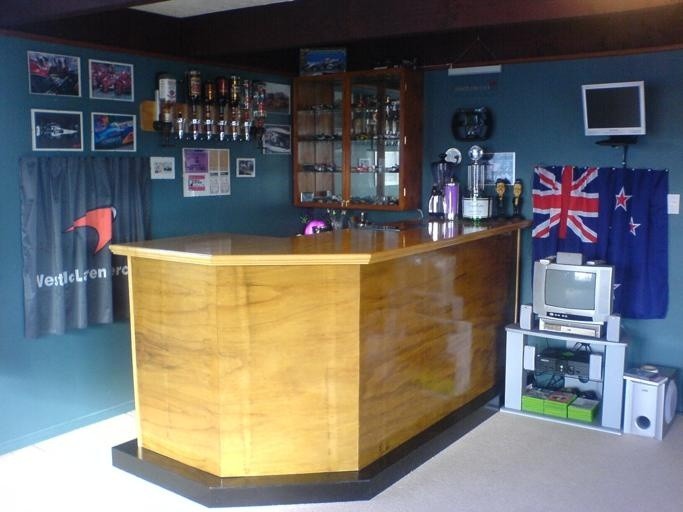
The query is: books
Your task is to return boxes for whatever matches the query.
[522,387,600,423]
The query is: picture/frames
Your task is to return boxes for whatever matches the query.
[30,108,84,151]
[27,49,81,97]
[90,111,137,153]
[88,58,135,103]
[261,81,291,154]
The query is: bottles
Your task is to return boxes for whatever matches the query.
[444,178,459,221]
[158,71,264,123]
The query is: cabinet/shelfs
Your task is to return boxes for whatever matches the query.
[503,325,627,435]
[291,68,422,212]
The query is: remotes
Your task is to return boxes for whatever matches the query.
[586,259,602,265]
[540,255,556,264]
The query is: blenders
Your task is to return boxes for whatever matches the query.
[429,153,453,216]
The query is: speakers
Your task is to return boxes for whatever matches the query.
[556,252,584,266]
[624,365,678,441]
[520,303,535,330]
[524,344,538,371]
[607,313,622,343]
[589,352,603,381]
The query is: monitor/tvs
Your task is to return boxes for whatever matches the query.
[582,81,647,146]
[533,259,615,325]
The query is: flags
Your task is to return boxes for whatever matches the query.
[532,166,668,318]
